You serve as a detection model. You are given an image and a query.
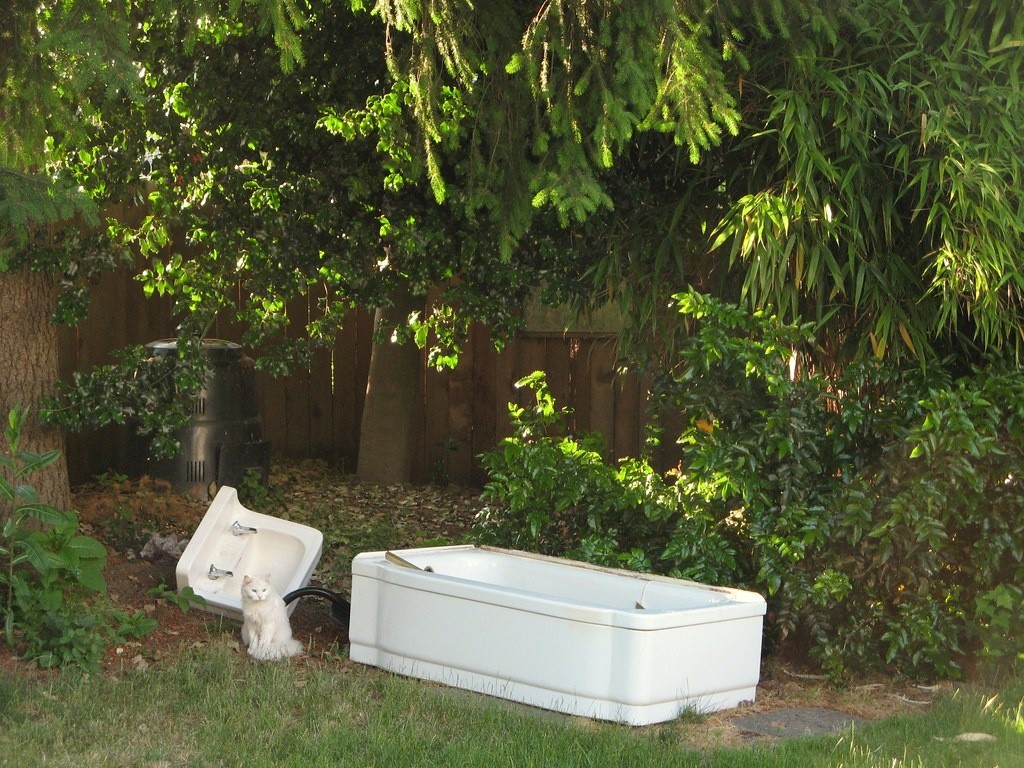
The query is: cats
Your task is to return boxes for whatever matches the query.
[241,573,305,662]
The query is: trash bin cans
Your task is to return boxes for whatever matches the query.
[143,338,261,497]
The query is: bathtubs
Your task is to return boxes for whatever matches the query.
[347,544,768,727]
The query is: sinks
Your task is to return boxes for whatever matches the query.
[194,508,324,626]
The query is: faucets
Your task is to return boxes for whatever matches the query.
[208,564,233,580]
[231,520,257,536]
